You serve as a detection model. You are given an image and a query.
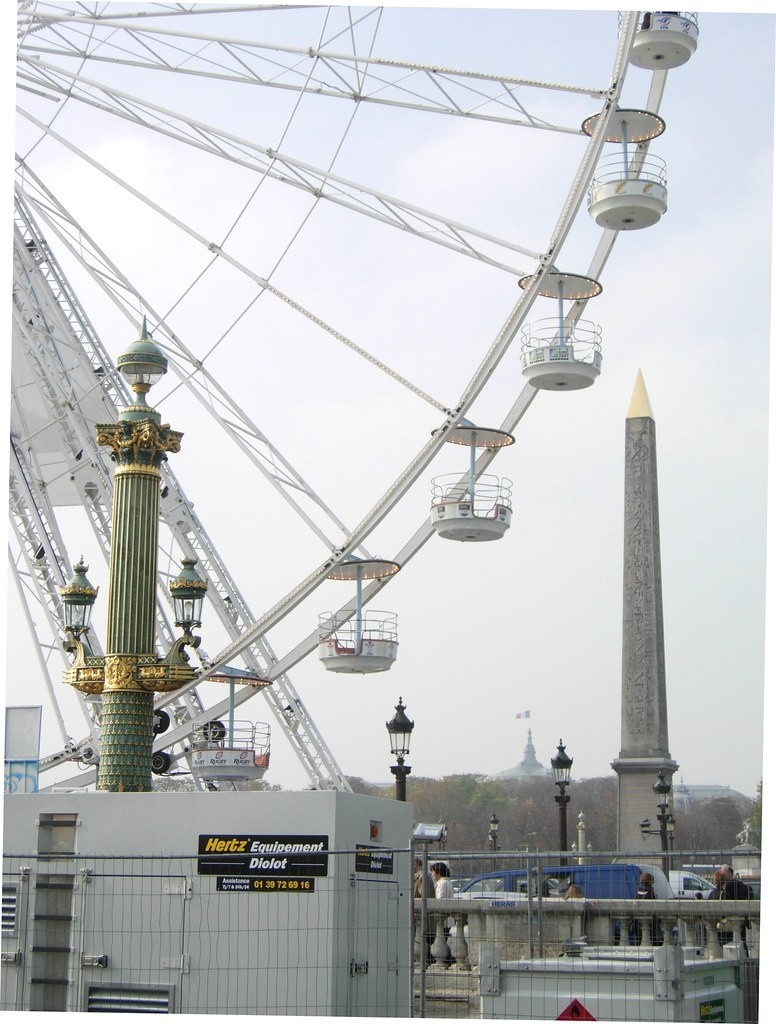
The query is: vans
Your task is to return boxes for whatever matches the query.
[456,865,674,946]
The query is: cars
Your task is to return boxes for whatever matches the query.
[669,864,761,946]
[448,878,558,898]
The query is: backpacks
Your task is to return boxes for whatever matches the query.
[735,880,753,900]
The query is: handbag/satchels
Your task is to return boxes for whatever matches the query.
[716,916,745,932]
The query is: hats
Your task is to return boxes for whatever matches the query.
[715,870,729,882]
[640,873,653,882]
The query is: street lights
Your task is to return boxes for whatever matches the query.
[639,771,672,882]
[551,738,574,879]
[487,811,500,871]
[59,315,208,793]
[385,695,415,802]
[571,809,593,865]
[667,811,676,871]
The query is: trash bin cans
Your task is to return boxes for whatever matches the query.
[586,914,616,947]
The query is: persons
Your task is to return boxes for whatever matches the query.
[693,892,708,956]
[703,864,751,960]
[413,857,457,970]
[563,883,587,901]
[628,872,665,946]
